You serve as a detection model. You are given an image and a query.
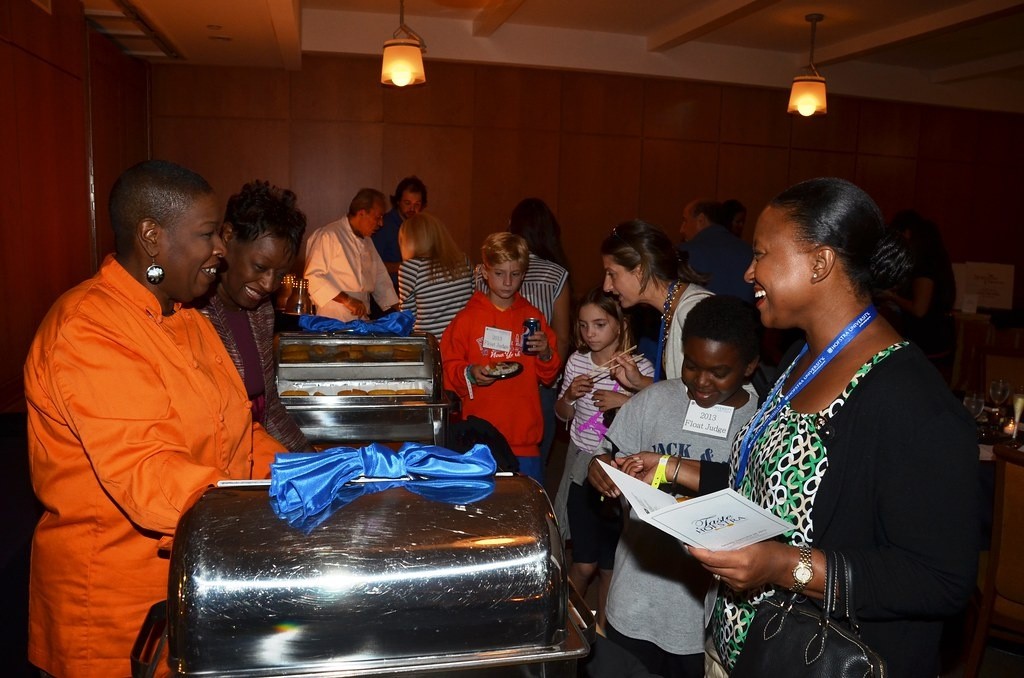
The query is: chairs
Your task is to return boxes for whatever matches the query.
[973,345,1024,405]
[960,444,1024,678]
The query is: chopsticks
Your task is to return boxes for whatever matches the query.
[589,345,644,379]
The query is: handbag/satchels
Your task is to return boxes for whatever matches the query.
[729,548,886,678]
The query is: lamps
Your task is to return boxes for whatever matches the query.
[788,13,829,118]
[380,0,427,86]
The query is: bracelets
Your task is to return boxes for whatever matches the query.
[462,364,478,401]
[344,295,353,306]
[671,454,682,485]
[652,453,671,488]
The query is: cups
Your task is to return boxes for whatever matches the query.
[989,378,1011,405]
[963,392,985,419]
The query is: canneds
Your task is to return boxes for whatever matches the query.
[522,318,541,355]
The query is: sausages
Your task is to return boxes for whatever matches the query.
[280,343,425,362]
[280,389,424,396]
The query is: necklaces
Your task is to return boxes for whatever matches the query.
[654,276,681,383]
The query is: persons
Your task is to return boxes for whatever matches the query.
[882,209,957,380]
[613,178,988,678]
[22,159,295,678]
[601,221,760,396]
[398,213,475,350]
[671,198,753,303]
[306,188,401,323]
[191,179,315,453]
[370,176,426,319]
[440,232,562,497]
[473,198,570,482]
[553,287,656,639]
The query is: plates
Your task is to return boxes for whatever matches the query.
[482,360,522,379]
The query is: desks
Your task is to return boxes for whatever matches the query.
[953,390,1024,462]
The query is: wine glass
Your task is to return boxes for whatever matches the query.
[1006,385,1024,449]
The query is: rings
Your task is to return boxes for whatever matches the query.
[711,572,721,582]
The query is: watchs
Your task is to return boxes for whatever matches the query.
[790,544,814,597]
[561,395,578,407]
